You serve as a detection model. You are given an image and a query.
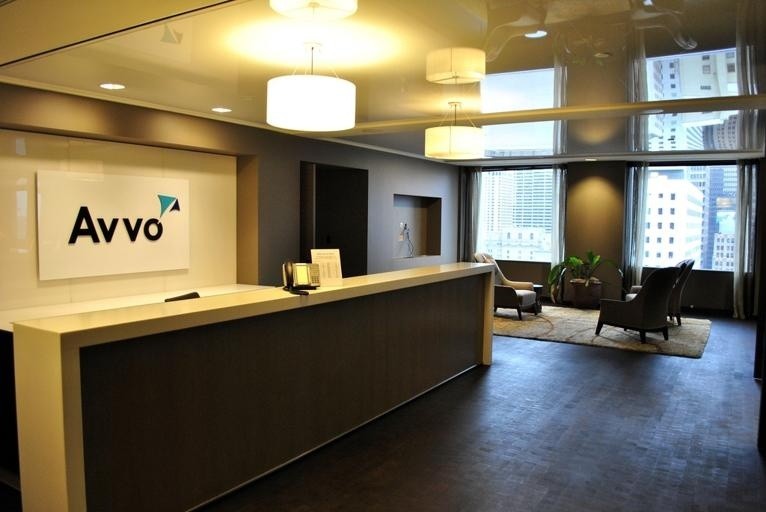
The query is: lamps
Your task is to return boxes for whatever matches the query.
[263,50,357,140]
[269,0,359,26]
[424,100,488,167]
[421,43,489,88]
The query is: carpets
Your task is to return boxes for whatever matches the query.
[491,298,713,359]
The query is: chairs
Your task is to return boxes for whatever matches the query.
[482,0,545,65]
[632,248,697,327]
[628,0,697,52]
[594,266,687,345]
[473,250,539,321]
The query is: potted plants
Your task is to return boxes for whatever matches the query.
[548,251,626,309]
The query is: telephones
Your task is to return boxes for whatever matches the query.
[282,260,320,290]
